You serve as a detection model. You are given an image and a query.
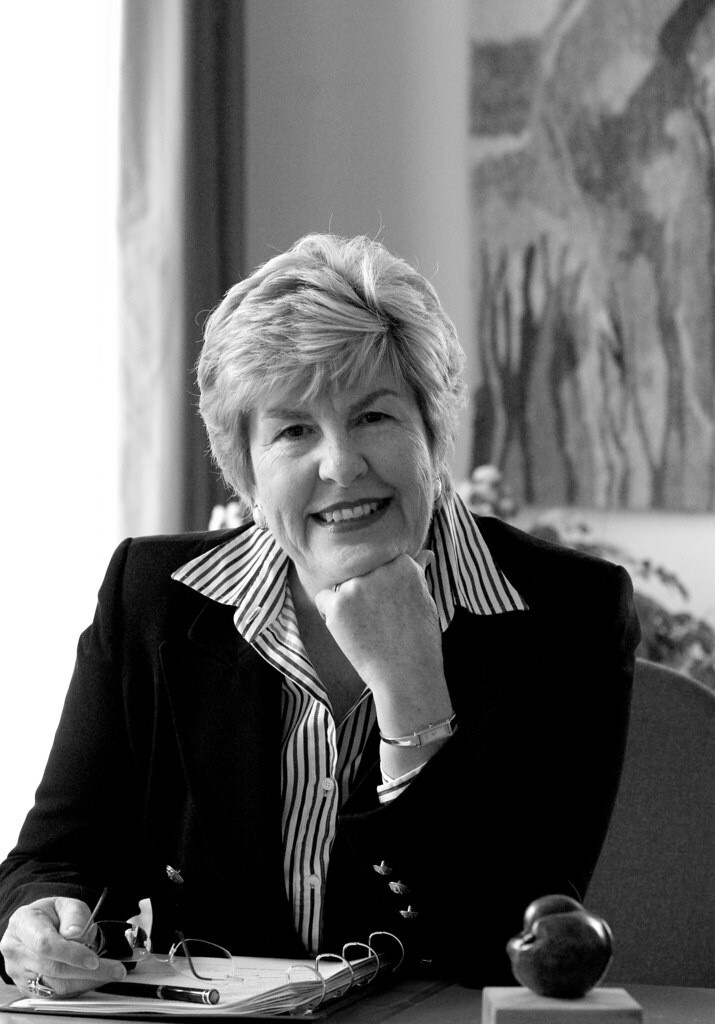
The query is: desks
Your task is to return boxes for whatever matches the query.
[0,975,715,1023]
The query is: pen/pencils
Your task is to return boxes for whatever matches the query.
[95,981,219,1005]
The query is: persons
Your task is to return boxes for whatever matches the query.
[0,233,643,1003]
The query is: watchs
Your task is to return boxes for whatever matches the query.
[379,713,458,748]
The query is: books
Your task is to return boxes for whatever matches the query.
[0,955,388,1024]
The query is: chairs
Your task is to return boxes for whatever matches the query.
[570,655,715,987]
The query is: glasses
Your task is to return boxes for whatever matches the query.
[76,887,244,983]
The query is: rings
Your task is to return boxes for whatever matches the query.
[27,973,54,998]
[333,584,341,592]
